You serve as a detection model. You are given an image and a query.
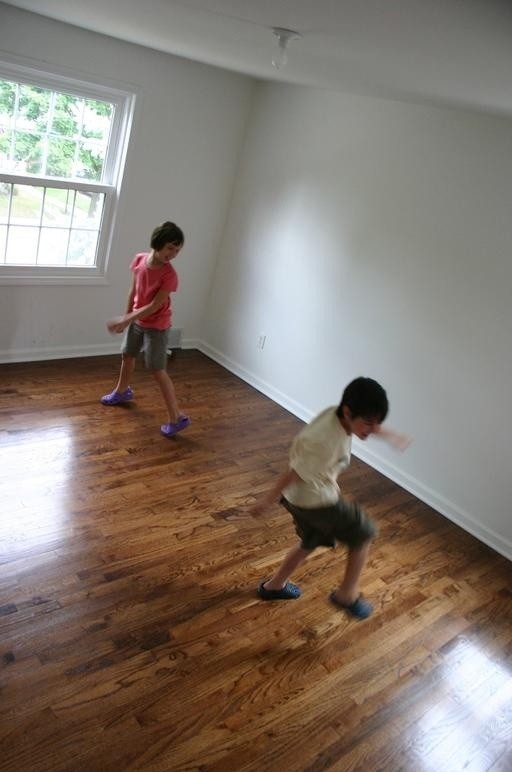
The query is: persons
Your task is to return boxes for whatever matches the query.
[243,376,418,621]
[98,221,190,438]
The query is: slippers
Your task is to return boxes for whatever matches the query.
[329,590,373,618]
[258,580,300,599]
[161,415,190,437]
[101,387,133,405]
